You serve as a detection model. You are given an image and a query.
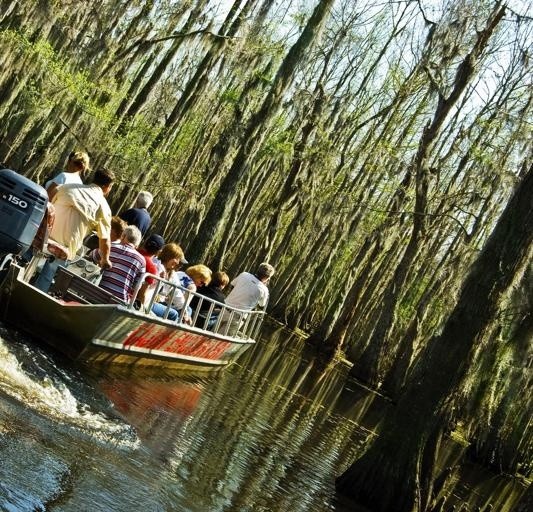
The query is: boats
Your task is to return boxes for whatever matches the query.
[0,168,265,372]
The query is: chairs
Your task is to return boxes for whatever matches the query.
[21,198,70,283]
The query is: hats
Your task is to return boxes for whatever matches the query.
[145,234,164,252]
[179,253,189,265]
